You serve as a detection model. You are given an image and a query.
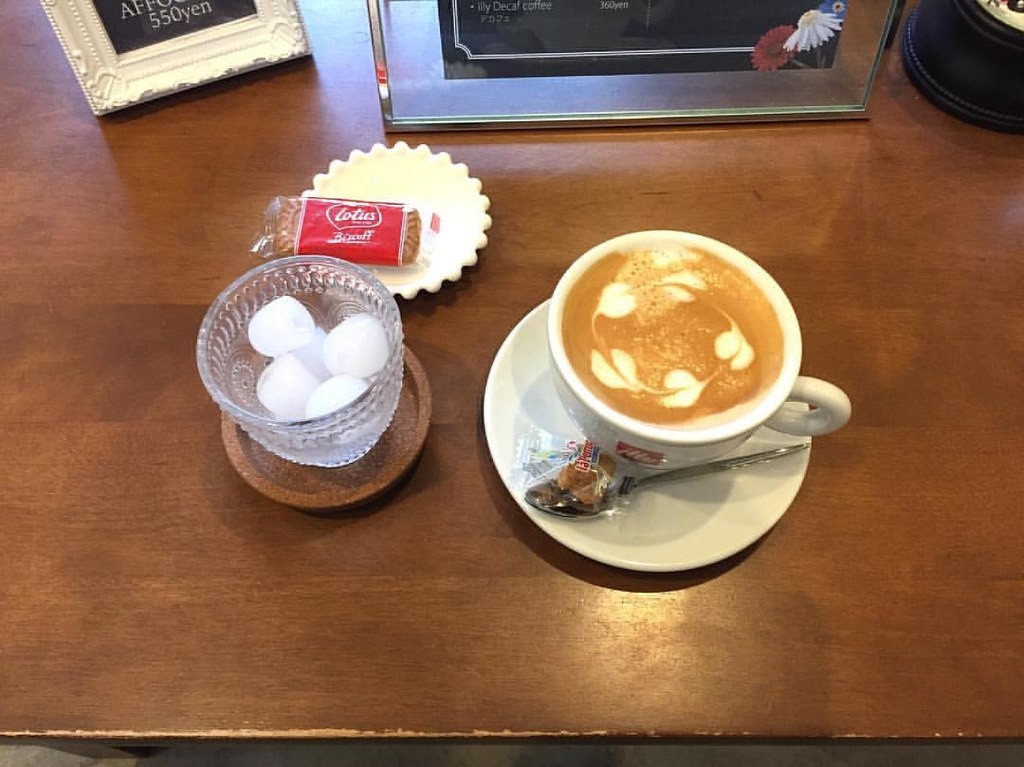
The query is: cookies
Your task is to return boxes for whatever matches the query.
[270,198,420,267]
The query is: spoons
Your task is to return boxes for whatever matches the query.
[524,443,811,518]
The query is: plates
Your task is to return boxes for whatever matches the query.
[482,298,812,573]
[221,342,434,510]
[300,141,494,300]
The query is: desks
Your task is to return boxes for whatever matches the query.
[3,2,1022,734]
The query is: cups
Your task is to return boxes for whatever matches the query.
[195,254,405,469]
[547,229,853,470]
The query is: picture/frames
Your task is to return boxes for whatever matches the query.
[44,1,315,120]
[362,1,908,129]
